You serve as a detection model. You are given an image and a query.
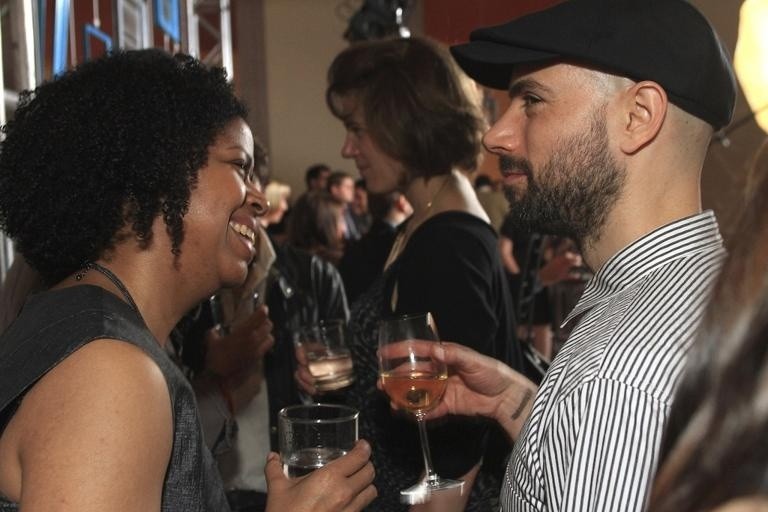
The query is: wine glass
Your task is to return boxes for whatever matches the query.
[377,312,467,497]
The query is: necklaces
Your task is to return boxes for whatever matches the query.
[393,170,452,257]
[72,261,143,314]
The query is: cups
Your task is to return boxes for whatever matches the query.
[278,405,360,482]
[207,293,260,336]
[291,320,356,394]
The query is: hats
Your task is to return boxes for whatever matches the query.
[448,0,737,128]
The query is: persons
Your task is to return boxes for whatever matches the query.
[538,232,593,350]
[328,173,357,229]
[500,208,552,364]
[356,183,371,225]
[472,175,511,233]
[306,162,328,200]
[1,255,274,465]
[339,190,412,296]
[279,200,349,336]
[292,36,521,512]
[375,2,738,511]
[1,51,268,512]
